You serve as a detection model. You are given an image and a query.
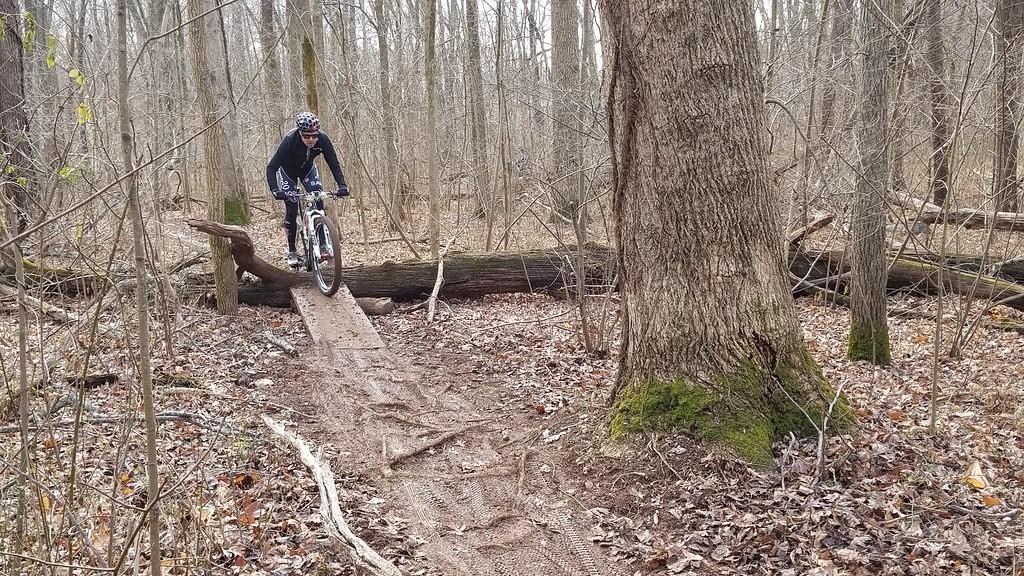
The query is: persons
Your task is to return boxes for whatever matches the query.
[266,112,350,267]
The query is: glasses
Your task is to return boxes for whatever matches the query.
[301,131,320,139]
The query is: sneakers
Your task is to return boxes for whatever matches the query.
[287,252,300,268]
[319,245,333,260]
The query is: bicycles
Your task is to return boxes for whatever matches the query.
[275,190,351,299]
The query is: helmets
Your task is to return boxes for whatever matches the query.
[296,112,320,132]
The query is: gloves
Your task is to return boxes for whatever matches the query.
[337,184,349,197]
[270,188,288,201]
